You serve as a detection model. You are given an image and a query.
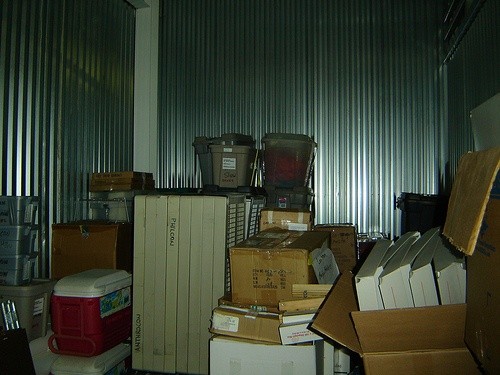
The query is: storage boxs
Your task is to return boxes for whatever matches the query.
[192,131,500,375]
[0,171,155,375]
